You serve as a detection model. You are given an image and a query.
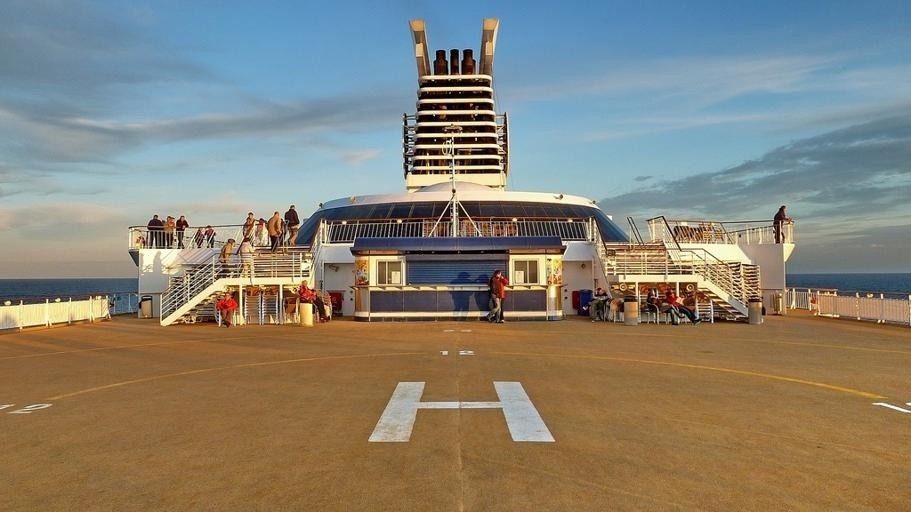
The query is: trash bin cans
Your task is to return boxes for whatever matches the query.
[623,295,638,326]
[579,289,593,316]
[139,296,153,318]
[748,295,763,324]
[299,297,313,327]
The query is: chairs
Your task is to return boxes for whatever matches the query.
[212,284,298,326]
[593,297,699,327]
[673,224,723,242]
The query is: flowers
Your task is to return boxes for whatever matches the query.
[293,286,317,302]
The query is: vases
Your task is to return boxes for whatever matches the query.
[300,303,314,327]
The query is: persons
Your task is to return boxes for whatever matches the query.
[215,291,238,328]
[772,205,792,244]
[582,287,609,323]
[297,279,329,323]
[218,238,236,279]
[666,287,702,326]
[646,288,686,326]
[146,204,301,254]
[237,237,256,279]
[486,270,502,324]
[497,273,509,323]
